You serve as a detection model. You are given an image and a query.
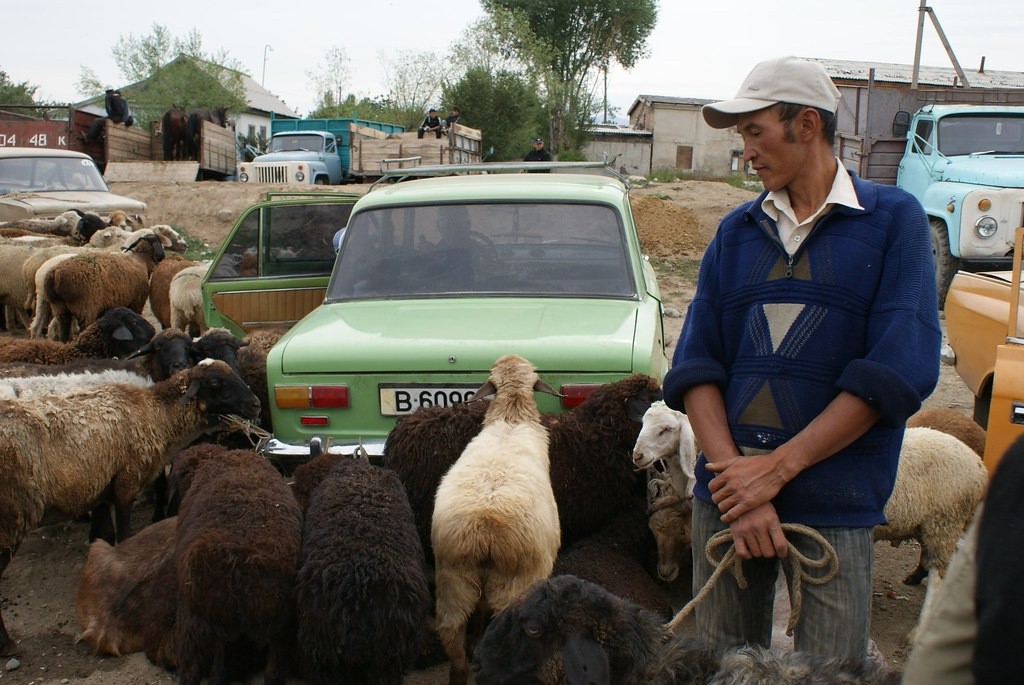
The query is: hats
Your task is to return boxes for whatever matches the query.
[701,56,841,130]
[535,138,542,144]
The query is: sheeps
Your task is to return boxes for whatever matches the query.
[0,208,993,684]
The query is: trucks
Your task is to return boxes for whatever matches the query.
[347,121,494,184]
[237,111,405,185]
[103,117,250,184]
[831,0,1024,309]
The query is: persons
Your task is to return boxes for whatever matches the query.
[661,55,942,664]
[441,106,461,136]
[519,138,552,173]
[418,109,442,138]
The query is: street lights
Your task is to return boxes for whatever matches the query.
[262,44,275,86]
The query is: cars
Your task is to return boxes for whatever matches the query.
[943,226,1024,475]
[0,147,148,229]
[201,172,669,476]
